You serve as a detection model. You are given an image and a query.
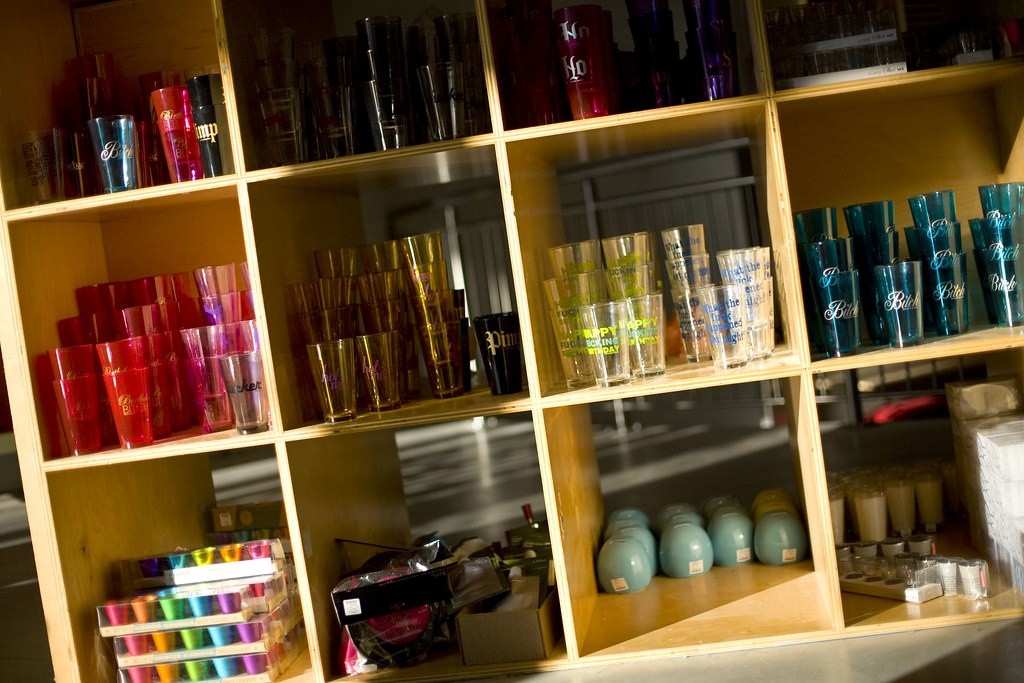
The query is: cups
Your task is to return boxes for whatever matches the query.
[541,225,775,388]
[295,232,524,422]
[794,177,1024,357]
[99,541,308,682]
[48,259,269,453]
[768,0,991,90]
[827,467,990,602]
[253,8,476,173]
[502,0,739,130]
[17,51,231,203]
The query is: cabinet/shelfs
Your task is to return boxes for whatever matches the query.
[0,0,1024,683]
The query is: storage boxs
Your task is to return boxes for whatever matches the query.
[331,546,557,670]
[946,377,1024,589]
